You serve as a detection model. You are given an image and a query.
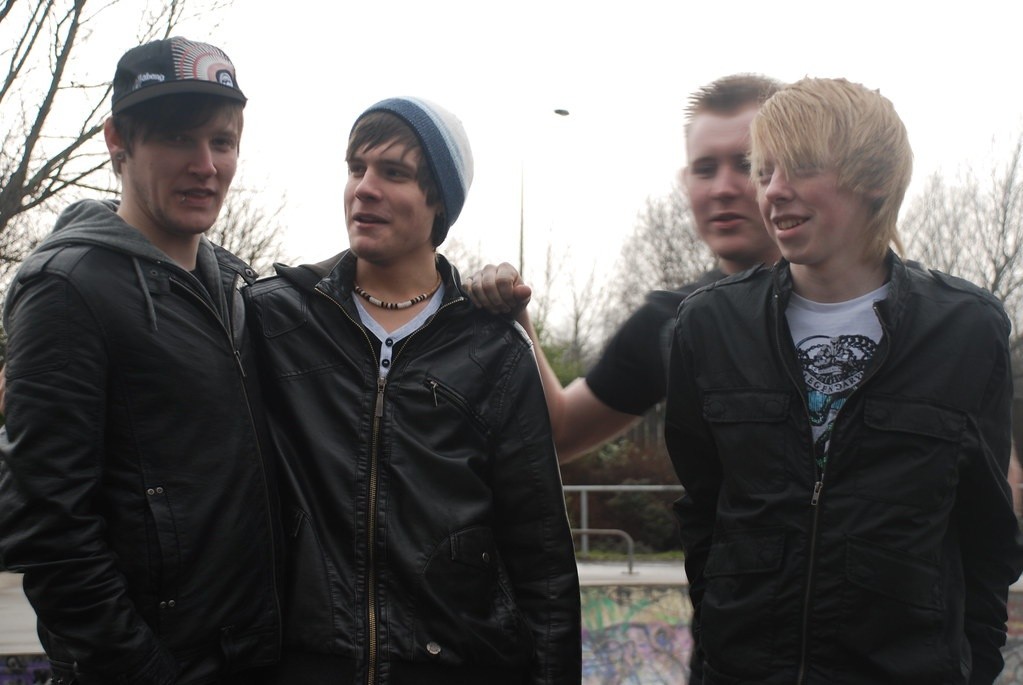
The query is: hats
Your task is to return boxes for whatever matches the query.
[110,36,247,114]
[348,96,475,228]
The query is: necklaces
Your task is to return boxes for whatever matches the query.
[351,271,442,310]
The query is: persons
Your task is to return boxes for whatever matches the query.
[657,77,1019,685]
[2,35,284,685]
[235,95,583,685]
[459,74,790,466]
